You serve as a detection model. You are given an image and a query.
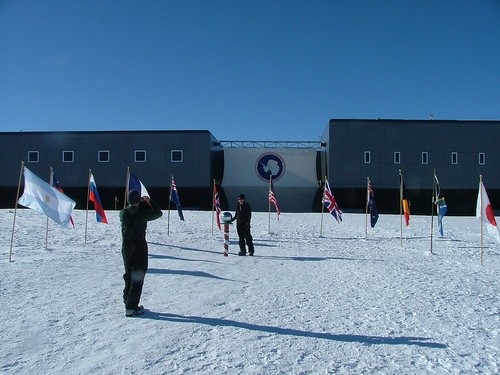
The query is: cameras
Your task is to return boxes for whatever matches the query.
[140,196,152,206]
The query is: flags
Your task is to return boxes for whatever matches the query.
[476,180,500,244]
[432,174,447,236]
[213,184,221,230]
[400,179,411,226]
[126,172,151,204]
[322,179,342,223]
[88,173,108,223]
[366,180,379,228]
[50,171,75,228]
[269,178,280,220]
[169,177,185,221]
[16,166,76,228]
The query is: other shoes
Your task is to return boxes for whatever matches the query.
[126,305,144,317]
[239,252,246,256]
[249,252,253,256]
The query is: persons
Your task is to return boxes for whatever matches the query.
[225,193,255,256]
[119,188,163,317]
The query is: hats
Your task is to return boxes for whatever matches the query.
[238,194,245,200]
[127,190,140,204]
